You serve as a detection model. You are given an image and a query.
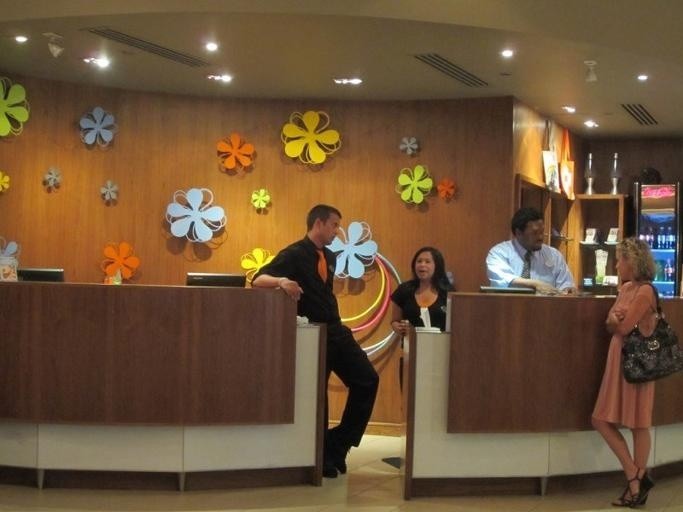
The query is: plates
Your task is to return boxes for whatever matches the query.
[579,241,599,245]
[604,242,617,246]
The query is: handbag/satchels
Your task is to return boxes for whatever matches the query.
[620,282,683,384]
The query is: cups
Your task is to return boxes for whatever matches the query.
[583,278,592,284]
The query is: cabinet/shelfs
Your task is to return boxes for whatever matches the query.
[574,194,631,295]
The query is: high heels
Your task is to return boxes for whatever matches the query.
[611,468,654,508]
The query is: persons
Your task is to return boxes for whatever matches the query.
[249,203,379,479]
[589,236,666,509]
[485,205,578,297]
[389,246,458,395]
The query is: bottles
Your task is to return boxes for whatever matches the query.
[653,257,674,281]
[582,152,597,196]
[609,152,620,195]
[639,225,674,249]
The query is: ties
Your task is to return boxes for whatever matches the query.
[314,249,328,284]
[521,252,531,279]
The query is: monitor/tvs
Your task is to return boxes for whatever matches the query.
[16,268,65,282]
[479,284,537,294]
[185,272,247,288]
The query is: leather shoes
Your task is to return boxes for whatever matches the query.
[323,423,347,478]
